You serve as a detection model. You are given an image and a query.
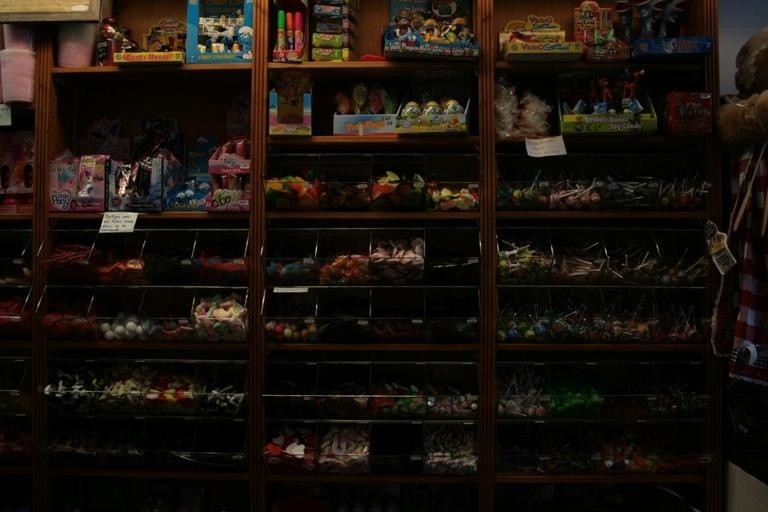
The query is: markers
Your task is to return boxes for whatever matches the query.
[294,11,304,51]
[277,10,285,50]
[286,12,294,50]
